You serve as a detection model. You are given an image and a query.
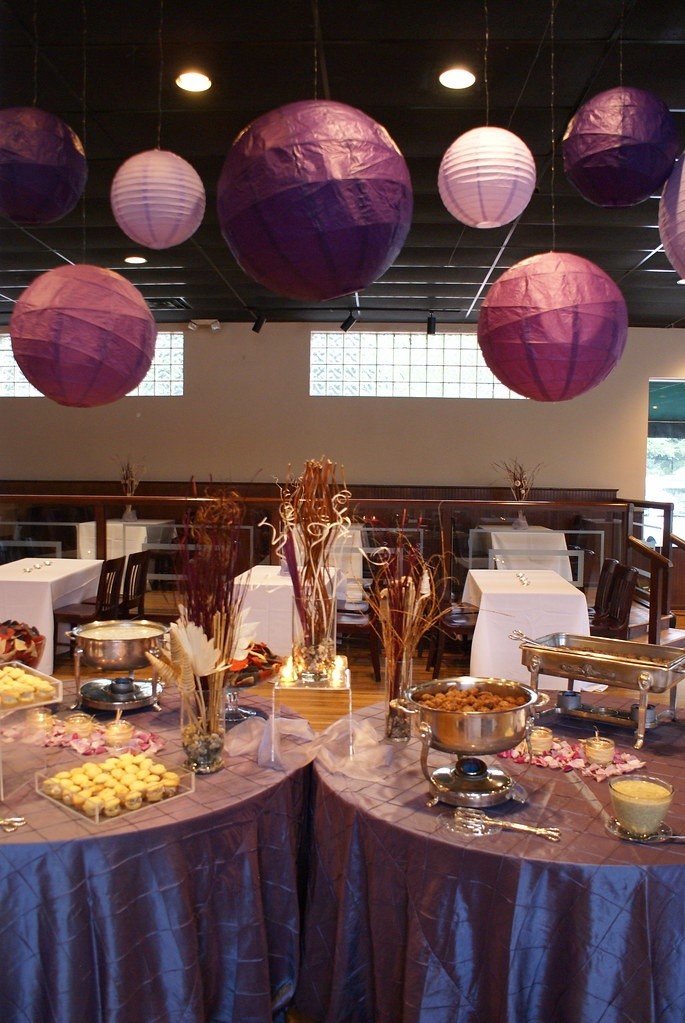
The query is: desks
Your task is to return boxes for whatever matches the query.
[468,526,574,582]
[0,557,105,676]
[462,568,609,693]
[78,519,177,595]
[223,565,348,684]
[281,523,370,601]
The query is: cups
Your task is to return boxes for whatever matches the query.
[105,720,134,747]
[524,726,553,755]
[585,736,615,769]
[609,774,674,836]
[65,713,93,738]
[27,708,52,731]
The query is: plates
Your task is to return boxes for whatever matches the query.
[605,816,673,844]
[436,810,502,835]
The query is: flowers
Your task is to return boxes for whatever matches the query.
[143,466,262,736]
[488,457,550,518]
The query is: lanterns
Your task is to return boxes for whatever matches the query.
[0,107,86,225]
[438,126,537,229]
[560,86,677,206]
[476,252,629,401]
[109,150,206,248]
[657,149,685,281]
[216,102,414,300]
[10,264,158,407]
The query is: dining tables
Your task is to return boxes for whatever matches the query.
[0,675,318,1023]
[310,689,685,1023]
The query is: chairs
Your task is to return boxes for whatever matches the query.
[0,505,639,682]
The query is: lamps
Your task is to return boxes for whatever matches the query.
[252,312,268,334]
[210,320,221,331]
[340,311,357,333]
[188,322,199,332]
[427,312,436,334]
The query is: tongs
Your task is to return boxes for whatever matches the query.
[453,806,561,842]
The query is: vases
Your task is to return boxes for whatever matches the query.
[512,513,528,530]
[289,596,334,685]
[120,510,139,523]
[384,658,413,742]
[180,688,228,776]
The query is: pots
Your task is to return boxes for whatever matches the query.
[390,676,550,754]
[64,619,171,671]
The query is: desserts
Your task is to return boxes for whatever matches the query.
[0,666,56,709]
[42,753,179,817]
